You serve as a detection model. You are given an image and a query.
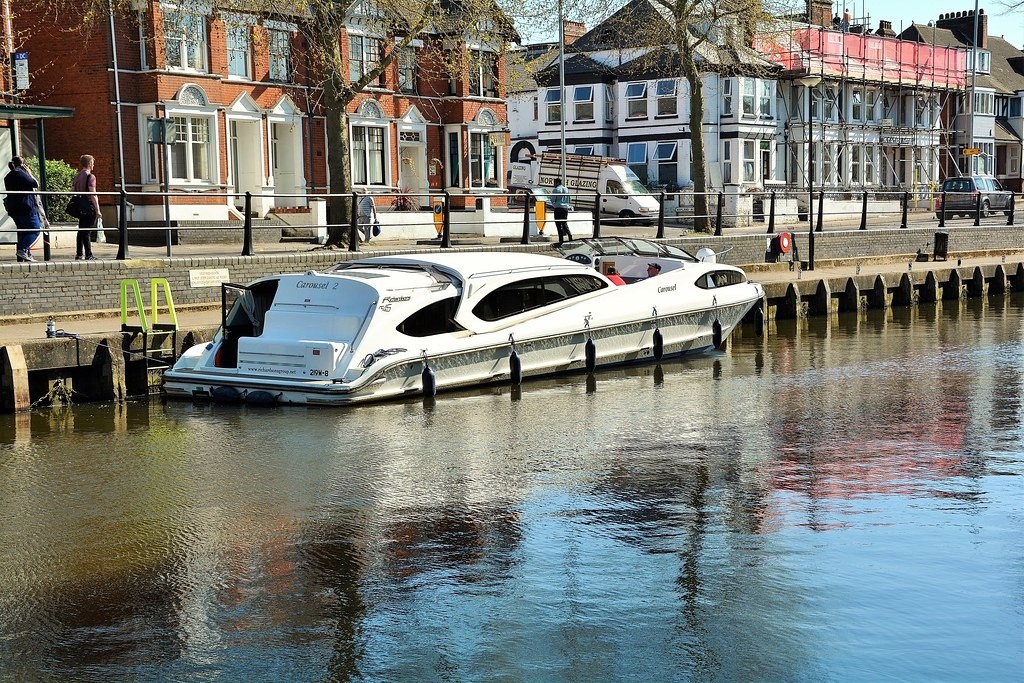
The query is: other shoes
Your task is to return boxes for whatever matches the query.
[86,255,98,260]
[75,254,85,260]
[16,249,38,263]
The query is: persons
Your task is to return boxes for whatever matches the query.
[647,262,661,276]
[4,156,42,261]
[551,178,573,242]
[358,187,377,239]
[607,266,626,287]
[833,8,850,33]
[72,155,102,260]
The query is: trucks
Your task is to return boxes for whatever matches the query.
[509,151,661,226]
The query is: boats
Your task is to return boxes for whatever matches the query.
[157,232,773,411]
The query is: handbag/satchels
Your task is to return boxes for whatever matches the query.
[65,195,93,220]
[373,220,380,236]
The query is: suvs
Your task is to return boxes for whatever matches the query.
[934,175,1015,220]
